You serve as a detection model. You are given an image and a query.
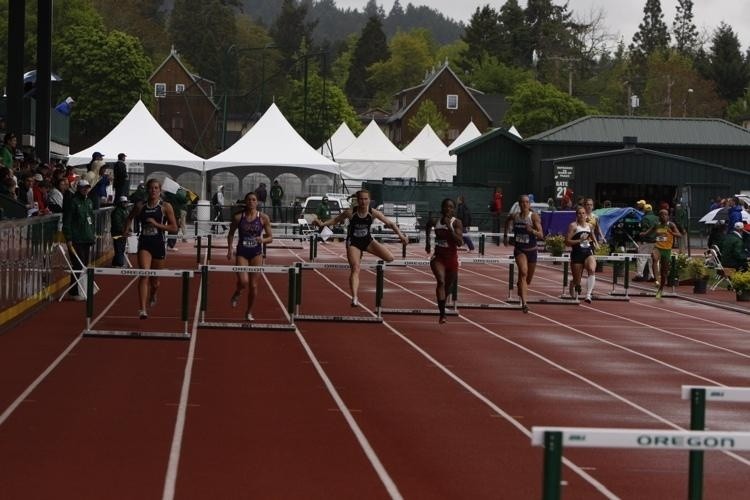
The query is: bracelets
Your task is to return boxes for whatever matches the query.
[503,234,507,237]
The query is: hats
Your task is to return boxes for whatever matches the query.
[92,152,104,156]
[78,180,91,188]
[734,222,744,230]
[637,199,652,212]
[121,196,127,202]
[118,154,127,157]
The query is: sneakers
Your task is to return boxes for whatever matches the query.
[167,239,188,251]
[570,281,575,298]
[632,276,655,282]
[439,316,447,324]
[69,295,84,301]
[655,281,662,299]
[244,314,255,321]
[352,297,358,306]
[138,309,147,320]
[233,301,237,307]
[522,305,529,313]
[584,297,592,304]
[150,294,157,307]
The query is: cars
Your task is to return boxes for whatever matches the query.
[372,204,421,242]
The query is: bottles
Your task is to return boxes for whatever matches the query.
[32,199,40,216]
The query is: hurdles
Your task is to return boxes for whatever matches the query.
[293,261,384,322]
[375,259,459,316]
[505,255,580,305]
[608,252,678,297]
[445,258,523,310]
[163,234,213,262]
[530,386,750,500]
[459,231,516,265]
[559,254,632,301]
[262,222,350,262]
[370,223,420,258]
[82,266,193,340]
[193,220,239,264]
[197,264,300,332]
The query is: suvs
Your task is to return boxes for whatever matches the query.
[508,203,557,250]
[300,197,350,231]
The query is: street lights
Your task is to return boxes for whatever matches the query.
[681,88,693,116]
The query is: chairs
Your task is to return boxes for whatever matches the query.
[704,249,736,292]
[298,218,315,247]
[712,245,721,255]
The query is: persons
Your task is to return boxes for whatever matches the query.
[315,189,409,307]
[548,189,689,304]
[256,184,267,214]
[0,134,198,319]
[317,197,331,221]
[211,185,228,233]
[289,195,308,224]
[710,195,750,290]
[271,180,284,223]
[426,199,463,324]
[228,191,273,321]
[456,196,475,253]
[503,195,544,314]
